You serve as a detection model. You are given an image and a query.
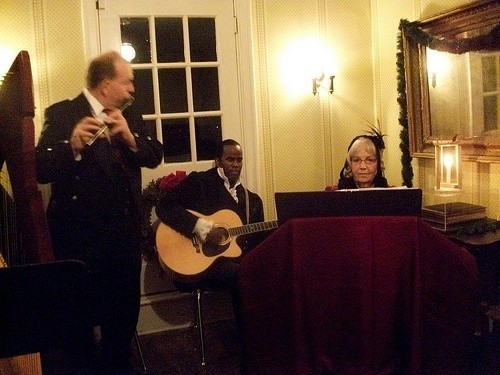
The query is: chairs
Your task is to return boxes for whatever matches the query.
[135,256,205,371]
[0,260,92,375]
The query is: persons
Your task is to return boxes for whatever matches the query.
[30,51,163,375]
[338,134,388,190]
[155,139,264,342]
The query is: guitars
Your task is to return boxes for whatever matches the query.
[155,208,280,283]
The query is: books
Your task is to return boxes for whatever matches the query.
[422,202,497,239]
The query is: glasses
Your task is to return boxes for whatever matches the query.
[351,156,377,165]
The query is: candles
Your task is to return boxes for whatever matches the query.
[446,163,451,184]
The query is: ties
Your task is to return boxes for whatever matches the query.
[102,108,126,174]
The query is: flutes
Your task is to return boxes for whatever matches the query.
[86,96,136,148]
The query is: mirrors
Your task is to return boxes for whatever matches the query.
[402,0,500,164]
